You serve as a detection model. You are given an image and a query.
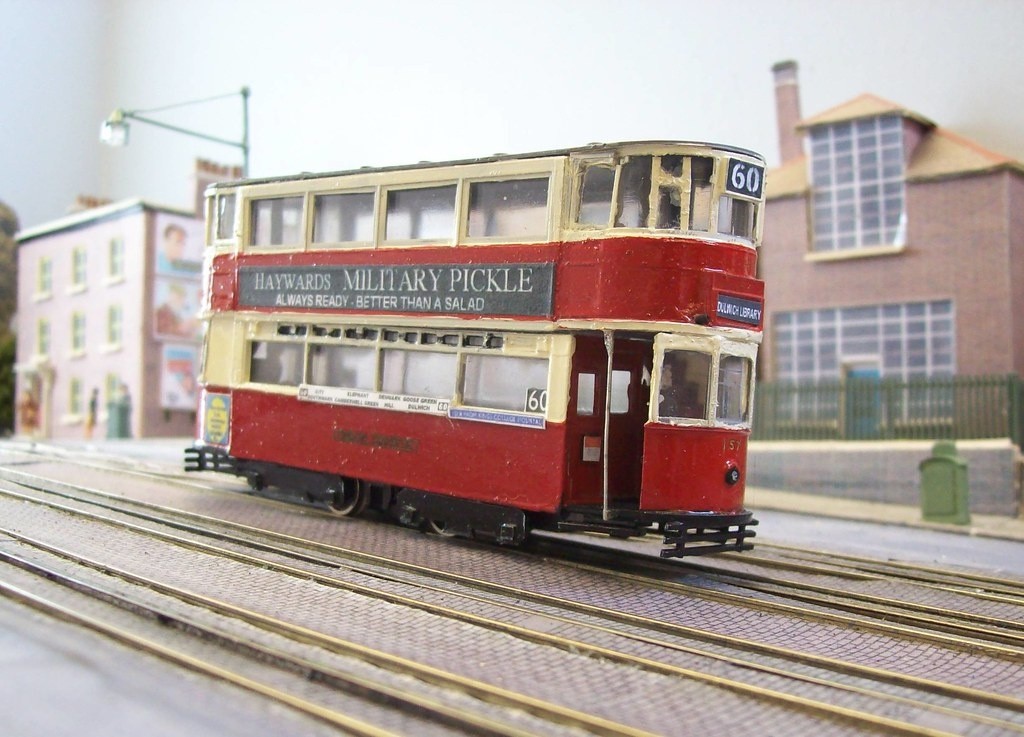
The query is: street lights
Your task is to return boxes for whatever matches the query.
[100,88,251,179]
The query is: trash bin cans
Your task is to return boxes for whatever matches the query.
[917,439,971,527]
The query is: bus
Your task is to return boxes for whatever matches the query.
[177,139,772,561]
